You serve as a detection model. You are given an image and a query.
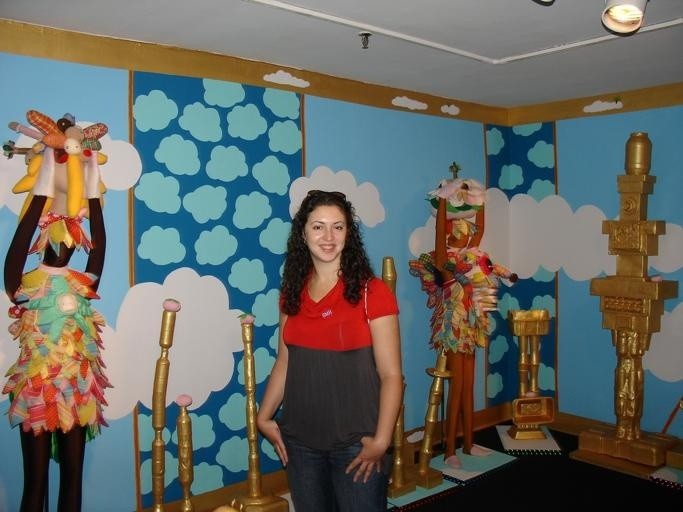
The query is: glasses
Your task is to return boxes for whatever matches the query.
[307,190,346,202]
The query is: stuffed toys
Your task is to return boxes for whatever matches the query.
[2,110,110,226]
[425,177,485,221]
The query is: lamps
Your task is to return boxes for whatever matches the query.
[600,0,647,35]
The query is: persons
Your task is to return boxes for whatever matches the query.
[419,196,499,469]
[1,147,115,510]
[253,189,404,510]
